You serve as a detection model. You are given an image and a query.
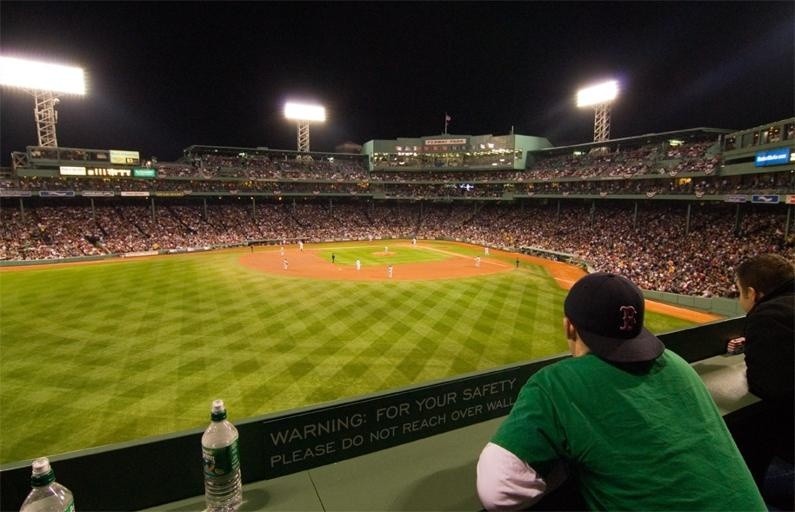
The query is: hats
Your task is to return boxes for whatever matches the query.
[565,272,666,363]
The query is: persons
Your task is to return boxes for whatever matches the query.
[726,252,795,512]
[477,272,770,512]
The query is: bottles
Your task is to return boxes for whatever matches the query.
[201,399,243,512]
[18,455,75,512]
